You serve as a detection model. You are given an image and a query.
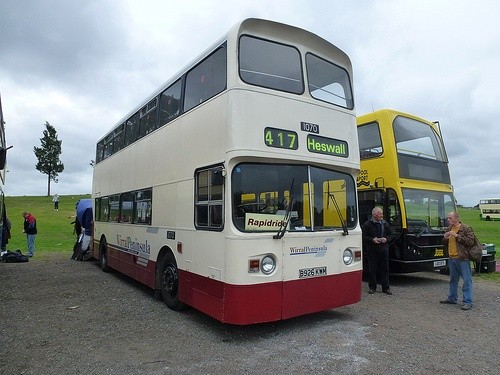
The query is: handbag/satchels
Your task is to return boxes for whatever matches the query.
[464,225,483,263]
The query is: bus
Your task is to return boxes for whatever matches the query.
[243,108,460,276]
[1,93,15,256]
[91,15,366,329]
[479,198,500,220]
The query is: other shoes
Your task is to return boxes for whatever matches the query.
[460,303,474,310]
[382,289,392,295]
[368,288,376,294]
[440,298,457,304]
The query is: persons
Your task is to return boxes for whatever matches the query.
[22,211,37,257]
[52,194,60,212]
[2,218,11,251]
[73,215,82,240]
[362,206,392,295]
[440,211,475,310]
